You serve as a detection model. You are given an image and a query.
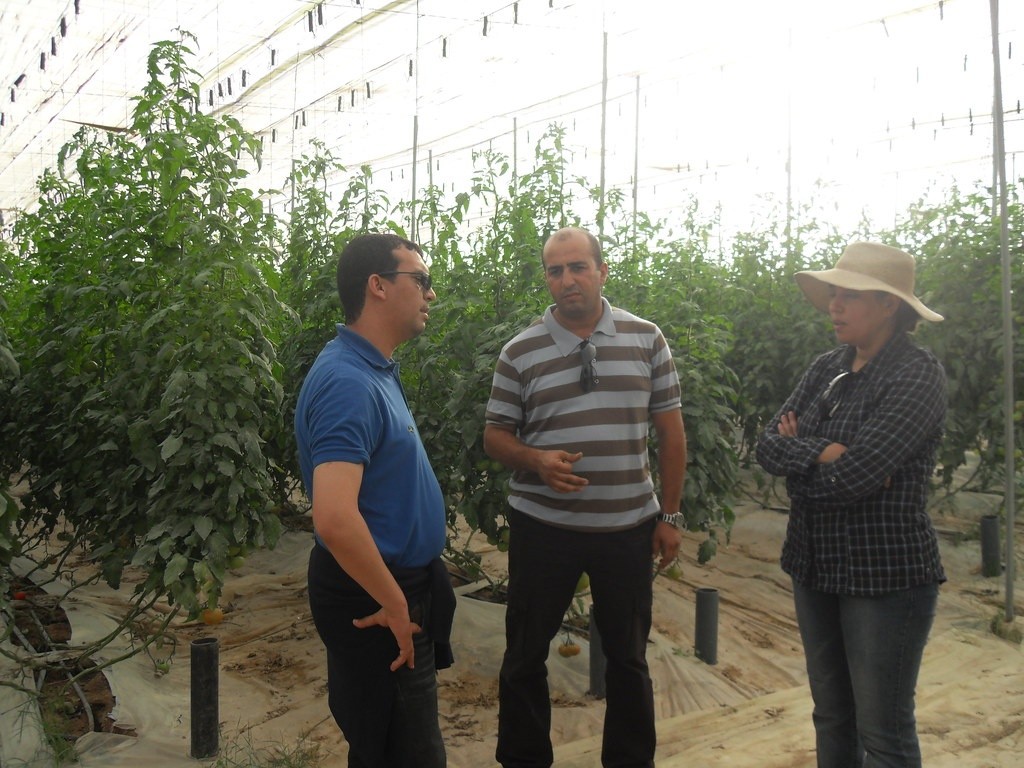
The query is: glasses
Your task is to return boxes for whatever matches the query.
[580,339,597,392]
[818,371,852,421]
[366,272,432,290]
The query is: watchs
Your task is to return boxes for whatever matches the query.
[658,512,684,528]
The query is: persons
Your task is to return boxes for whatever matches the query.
[295,235,449,767]
[754,244,949,768]
[480,225,686,768]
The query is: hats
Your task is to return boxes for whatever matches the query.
[794,242,945,332]
